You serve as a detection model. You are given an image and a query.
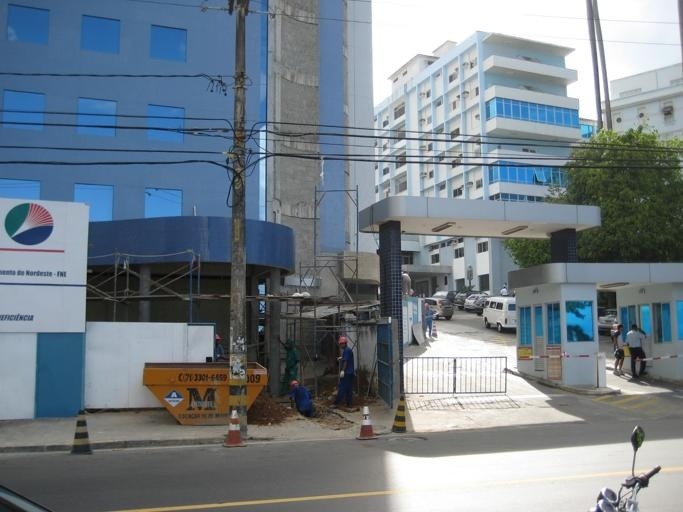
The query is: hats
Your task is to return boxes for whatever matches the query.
[420,289,515,332]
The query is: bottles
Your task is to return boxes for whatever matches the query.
[291,380,299,384]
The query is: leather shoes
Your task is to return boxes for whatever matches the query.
[339,370,345,376]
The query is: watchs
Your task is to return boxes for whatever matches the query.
[589,426,661,512]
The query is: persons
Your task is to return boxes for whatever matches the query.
[611,325,625,376]
[331,337,354,408]
[424,303,433,337]
[289,380,313,418]
[214,335,224,362]
[278,339,302,396]
[626,324,648,378]
[501,287,507,296]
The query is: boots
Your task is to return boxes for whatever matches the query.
[597,303,617,341]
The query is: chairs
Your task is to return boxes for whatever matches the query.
[613,369,624,376]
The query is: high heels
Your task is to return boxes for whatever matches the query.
[355,404,377,439]
[430,319,437,336]
[69,410,91,455]
[390,396,407,433]
[223,408,244,448]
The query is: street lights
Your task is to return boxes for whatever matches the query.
[623,344,631,356]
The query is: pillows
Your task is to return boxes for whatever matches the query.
[286,338,295,345]
[339,337,348,344]
[425,302,429,308]
[215,334,219,340]
